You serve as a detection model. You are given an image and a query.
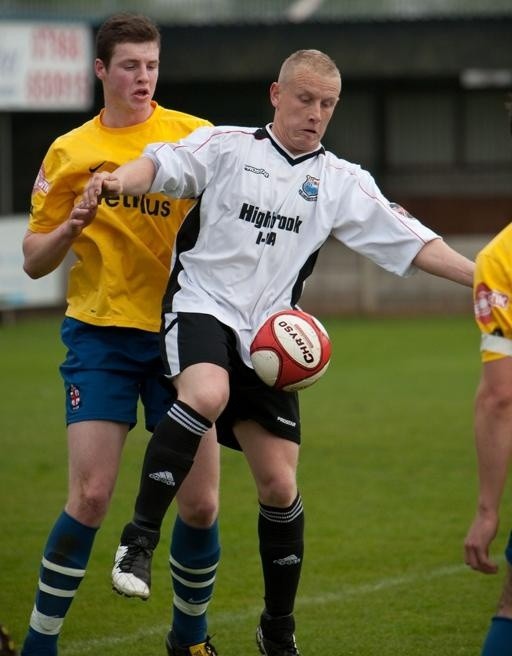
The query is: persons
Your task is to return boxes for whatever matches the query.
[81,48,478,656]
[19,13,231,656]
[460,219,512,656]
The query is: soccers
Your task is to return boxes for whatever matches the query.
[249,310,332,392]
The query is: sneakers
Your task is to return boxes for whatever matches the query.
[109,522,160,600]
[165,630,217,656]
[254,610,300,656]
